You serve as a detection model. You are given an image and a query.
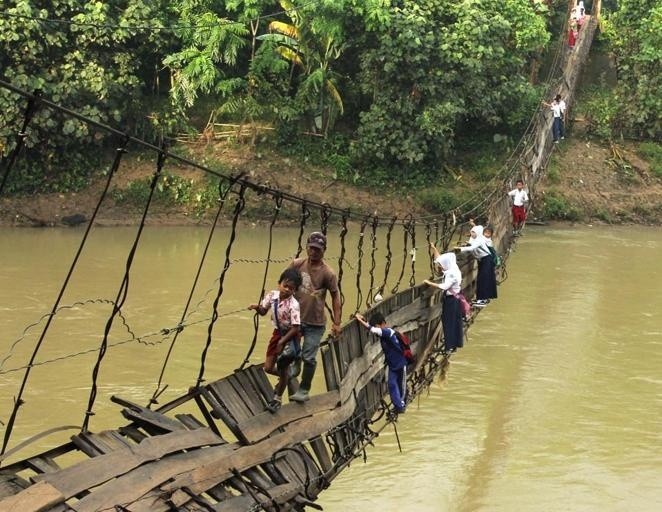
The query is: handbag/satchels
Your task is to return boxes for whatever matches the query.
[572,31,579,39]
[449,288,471,315]
[275,336,302,371]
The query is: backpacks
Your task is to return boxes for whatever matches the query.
[477,242,502,267]
[385,328,415,365]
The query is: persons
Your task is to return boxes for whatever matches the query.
[506,180,529,236]
[423,242,463,354]
[353,312,408,414]
[568,0,586,50]
[248,270,302,415]
[454,224,497,307]
[284,232,341,402]
[542,95,567,144]
[469,217,501,266]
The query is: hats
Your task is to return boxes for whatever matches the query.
[305,230,327,252]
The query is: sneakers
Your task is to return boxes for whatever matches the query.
[389,405,406,414]
[472,299,487,308]
[264,395,281,413]
[288,388,310,402]
[437,346,457,355]
[551,136,564,144]
